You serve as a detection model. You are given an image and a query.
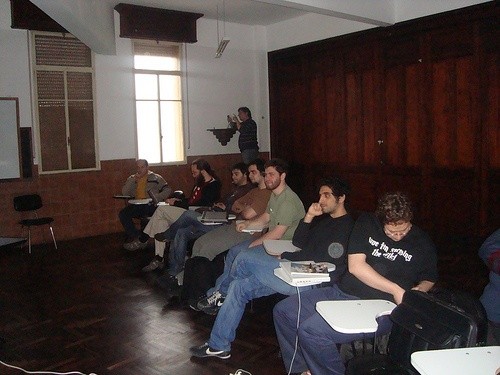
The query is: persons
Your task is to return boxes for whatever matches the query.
[190,174,355,359]
[119,159,305,314]
[273,192,438,375]
[227,107,259,163]
[478,230,500,347]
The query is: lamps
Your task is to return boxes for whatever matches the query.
[214,0,230,58]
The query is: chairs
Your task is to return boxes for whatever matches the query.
[13,193,58,254]
[346,289,480,375]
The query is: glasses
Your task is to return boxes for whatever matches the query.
[384,224,411,237]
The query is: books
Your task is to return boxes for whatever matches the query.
[279,261,332,283]
[201,211,228,223]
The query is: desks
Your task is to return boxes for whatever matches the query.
[274,261,336,287]
[236,220,263,232]
[315,298,397,336]
[196,213,236,226]
[410,345,500,375]
[112,195,132,199]
[128,198,153,205]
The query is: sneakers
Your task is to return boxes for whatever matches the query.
[124,238,152,250]
[198,292,226,314]
[190,341,231,360]
[142,259,165,272]
